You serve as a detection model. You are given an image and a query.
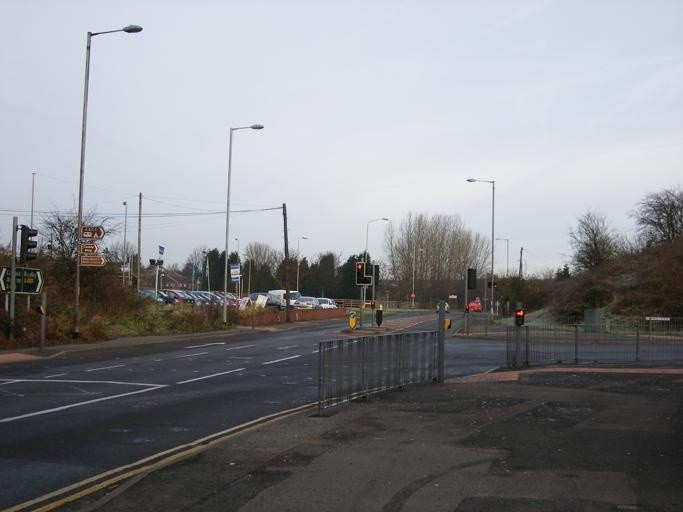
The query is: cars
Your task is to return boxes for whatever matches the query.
[139,290,337,309]
[435,301,449,313]
[467,301,480,311]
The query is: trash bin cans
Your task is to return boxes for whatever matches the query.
[584,308,604,332]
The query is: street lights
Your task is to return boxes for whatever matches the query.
[219,124,263,327]
[466,179,494,322]
[200,249,211,291]
[411,246,424,309]
[363,217,388,311]
[148,259,162,300]
[296,236,308,290]
[74,24,143,340]
[232,238,239,295]
[495,237,508,277]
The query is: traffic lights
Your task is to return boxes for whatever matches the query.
[20,225,37,261]
[516,310,524,325]
[357,263,371,284]
[488,281,497,289]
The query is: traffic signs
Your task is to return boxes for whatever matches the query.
[1,266,44,294]
